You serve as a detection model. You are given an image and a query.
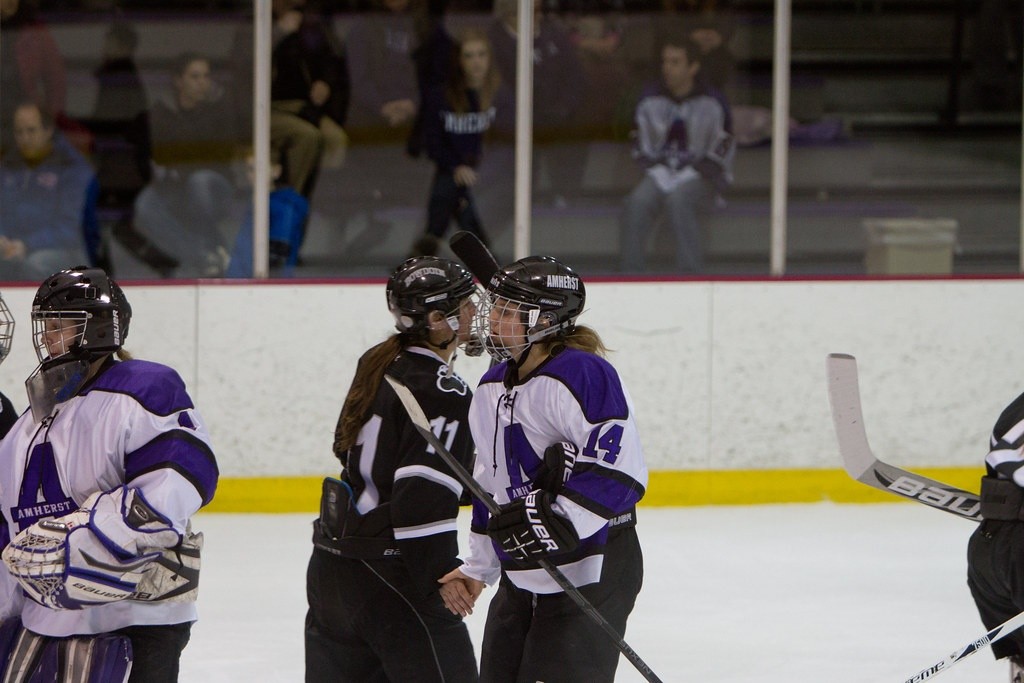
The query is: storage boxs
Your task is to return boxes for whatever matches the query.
[863,213,960,276]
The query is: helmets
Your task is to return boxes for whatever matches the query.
[487,256,587,342]
[386,256,478,334]
[32,265,133,392]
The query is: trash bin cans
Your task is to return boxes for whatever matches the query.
[864,217,960,275]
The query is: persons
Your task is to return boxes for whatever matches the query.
[0,0,755,280]
[0,265,220,683]
[968,393,1024,683]
[438,257,647,683]
[304,252,492,683]
[57,24,180,281]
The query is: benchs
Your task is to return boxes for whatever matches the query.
[39,28,1022,255]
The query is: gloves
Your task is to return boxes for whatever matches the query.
[486,491,577,568]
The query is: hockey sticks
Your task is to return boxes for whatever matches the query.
[901,609,1024,683]
[447,228,502,290]
[822,348,984,521]
[382,367,661,682]
